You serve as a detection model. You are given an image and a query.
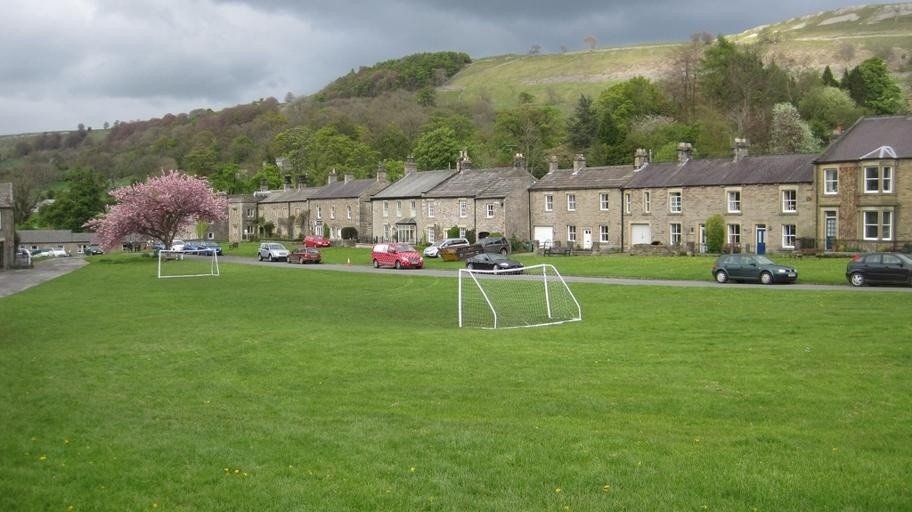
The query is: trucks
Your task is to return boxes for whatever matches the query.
[371,242,424,269]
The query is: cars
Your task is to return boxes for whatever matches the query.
[153,239,222,256]
[845,251,912,287]
[85,247,104,255]
[17,247,67,259]
[711,252,798,284]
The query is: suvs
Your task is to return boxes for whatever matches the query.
[303,235,331,248]
[286,248,321,264]
[257,242,291,261]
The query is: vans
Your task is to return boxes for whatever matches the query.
[472,237,511,256]
[423,238,469,257]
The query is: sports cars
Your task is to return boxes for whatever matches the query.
[465,253,524,275]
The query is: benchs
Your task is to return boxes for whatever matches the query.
[544,249,571,256]
